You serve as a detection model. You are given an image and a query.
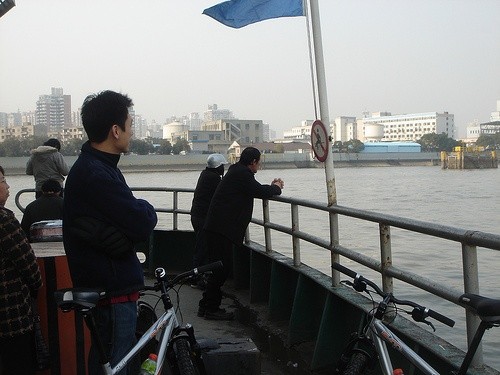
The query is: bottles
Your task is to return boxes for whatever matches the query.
[137,353,159,375]
[393,368,402,375]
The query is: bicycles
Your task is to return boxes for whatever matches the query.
[54,259,226,375]
[329,262,500,375]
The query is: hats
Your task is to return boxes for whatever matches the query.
[42,178,61,192]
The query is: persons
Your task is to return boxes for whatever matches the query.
[25,139,69,200]
[0,162,46,375]
[17,180,65,240]
[189,154,229,289]
[64,91,157,375]
[196,146,285,320]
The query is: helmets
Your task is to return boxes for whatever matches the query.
[206,153,228,168]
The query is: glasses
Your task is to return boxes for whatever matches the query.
[1,179,7,183]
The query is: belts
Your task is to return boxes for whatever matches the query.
[100,293,139,304]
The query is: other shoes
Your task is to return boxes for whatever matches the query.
[198,308,225,317]
[191,283,205,288]
[205,311,234,320]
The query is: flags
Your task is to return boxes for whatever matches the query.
[203,0,306,28]
